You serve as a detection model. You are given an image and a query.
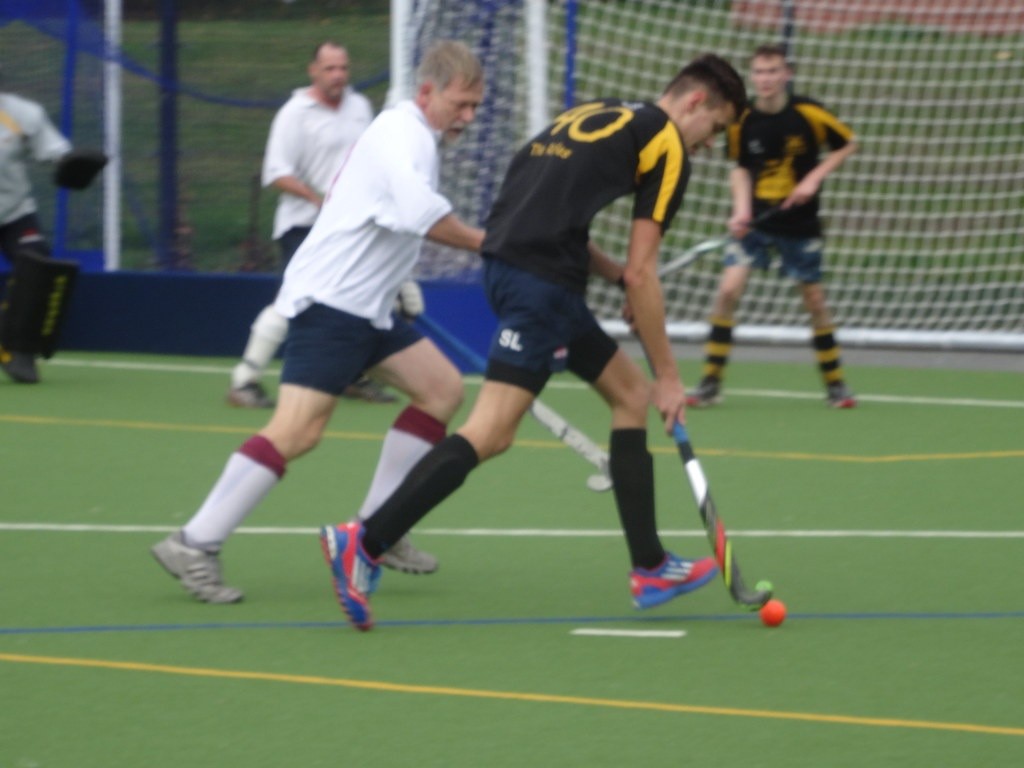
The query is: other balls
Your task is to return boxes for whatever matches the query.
[760,598,787,627]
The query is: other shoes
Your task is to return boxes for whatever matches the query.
[824,390,855,407]
[224,381,274,409]
[342,374,396,404]
[0,345,40,384]
[683,382,722,406]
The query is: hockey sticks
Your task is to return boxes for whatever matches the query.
[615,205,784,294]
[670,418,773,612]
[394,292,617,496]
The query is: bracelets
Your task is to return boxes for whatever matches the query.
[617,277,627,293]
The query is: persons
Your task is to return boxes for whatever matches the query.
[227,39,401,409]
[151,38,486,602]
[322,56,746,631]
[0,92,108,387]
[683,47,860,407]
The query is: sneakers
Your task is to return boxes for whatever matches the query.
[628,550,719,610]
[380,533,439,573]
[150,529,244,604]
[319,518,387,632]
